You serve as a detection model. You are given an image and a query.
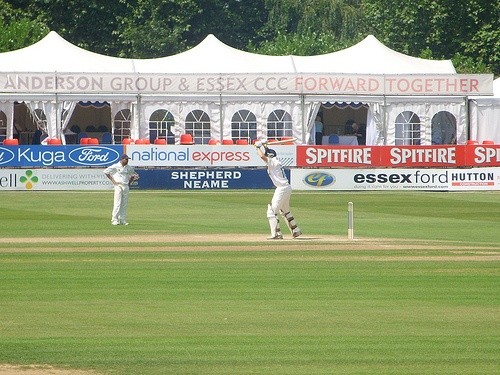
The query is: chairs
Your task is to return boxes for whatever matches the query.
[268,138,277,142]
[180,133,193,145]
[110,128,119,141]
[466,140,479,145]
[154,138,166,144]
[70,125,81,134]
[32,130,42,145]
[79,137,100,144]
[252,139,261,145]
[328,134,340,146]
[79,132,87,138]
[3,138,19,145]
[121,138,134,144]
[97,125,109,132]
[85,125,97,132]
[46,138,62,145]
[280,138,294,145]
[208,139,220,145]
[236,139,248,145]
[483,140,494,145]
[222,139,234,145]
[102,133,112,144]
[135,138,150,144]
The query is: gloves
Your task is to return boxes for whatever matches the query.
[261,139,267,144]
[254,141,262,148]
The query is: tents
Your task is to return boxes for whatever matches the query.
[0,30,500,145]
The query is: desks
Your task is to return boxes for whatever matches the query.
[77,131,110,144]
[20,130,34,145]
[322,135,359,145]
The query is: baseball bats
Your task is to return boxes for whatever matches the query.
[254,138,297,147]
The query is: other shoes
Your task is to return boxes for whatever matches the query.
[112,221,119,226]
[292,232,301,238]
[118,219,128,226]
[267,234,282,240]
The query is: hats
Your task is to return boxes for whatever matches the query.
[263,149,277,158]
[121,154,131,159]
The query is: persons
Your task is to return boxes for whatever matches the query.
[254,139,302,240]
[104,154,134,226]
[315,115,324,146]
[349,123,366,145]
